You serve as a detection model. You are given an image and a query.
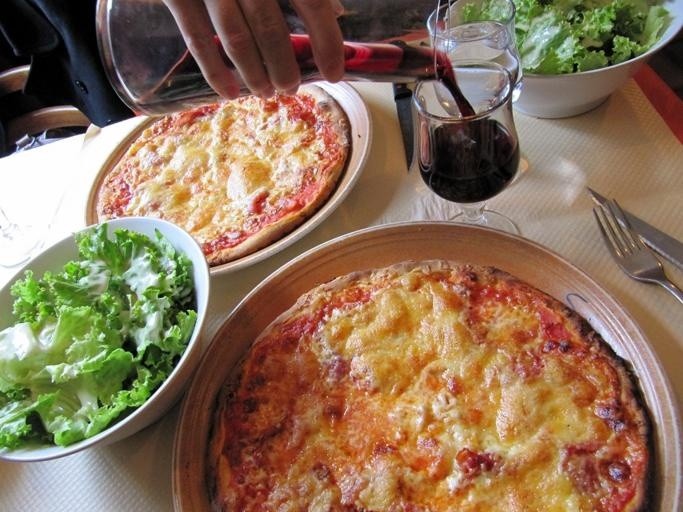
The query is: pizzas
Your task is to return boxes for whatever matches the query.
[96,81,350,265]
[207,259,654,512]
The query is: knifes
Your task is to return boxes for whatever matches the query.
[586,185,682,269]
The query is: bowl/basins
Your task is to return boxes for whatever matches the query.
[443,0,682,120]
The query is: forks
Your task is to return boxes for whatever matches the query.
[590,198,682,303]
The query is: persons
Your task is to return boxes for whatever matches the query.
[0,0,346,143]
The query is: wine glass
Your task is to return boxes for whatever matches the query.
[411,62,524,239]
[424,0,530,177]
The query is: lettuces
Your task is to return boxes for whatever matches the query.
[460,0,674,76]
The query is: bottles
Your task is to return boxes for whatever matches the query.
[95,0,455,117]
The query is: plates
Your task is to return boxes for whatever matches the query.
[84,78,372,279]
[168,218,682,511]
